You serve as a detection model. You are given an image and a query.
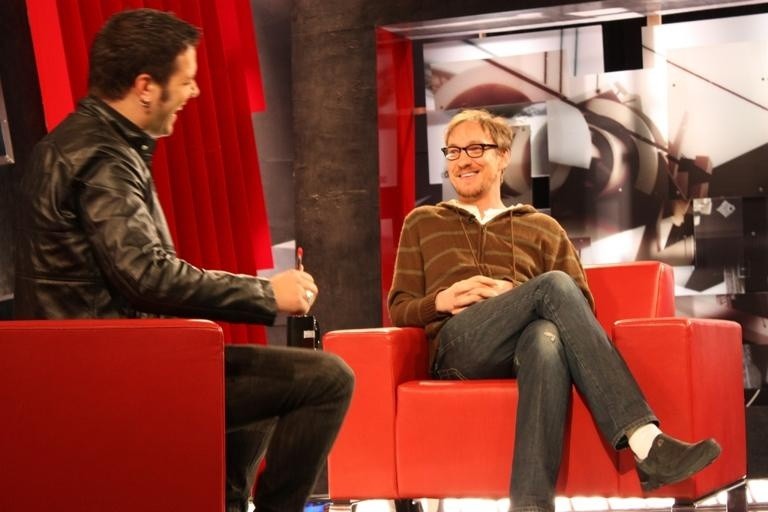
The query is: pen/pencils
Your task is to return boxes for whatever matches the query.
[296,248,303,272]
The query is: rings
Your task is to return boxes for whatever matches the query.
[304,289,313,302]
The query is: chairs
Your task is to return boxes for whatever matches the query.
[322,259,749,512]
[1,318,227,512]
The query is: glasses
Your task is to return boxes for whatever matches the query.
[440,143,498,161]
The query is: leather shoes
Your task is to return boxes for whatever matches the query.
[633,432,721,493]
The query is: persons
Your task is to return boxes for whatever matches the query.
[388,107,721,512]
[11,9,356,511]
